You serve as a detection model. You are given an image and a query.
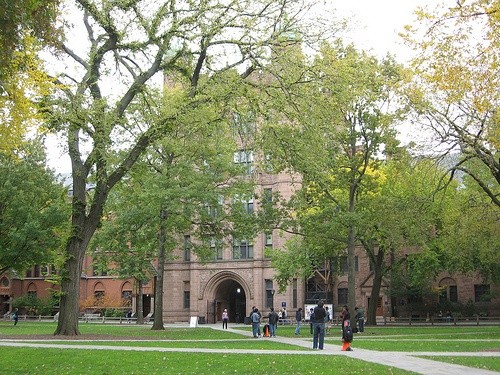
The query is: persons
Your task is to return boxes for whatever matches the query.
[353,306,365,334]
[341,319,353,351]
[277,307,288,325]
[313,299,327,351]
[341,305,350,339]
[13,308,19,327]
[263,307,280,339]
[127,310,132,323]
[323,306,331,335]
[295,307,303,335]
[308,308,314,334]
[250,306,263,338]
[222,308,229,329]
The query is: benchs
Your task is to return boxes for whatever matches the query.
[82,314,100,320]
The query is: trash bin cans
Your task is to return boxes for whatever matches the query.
[244,317,251,325]
